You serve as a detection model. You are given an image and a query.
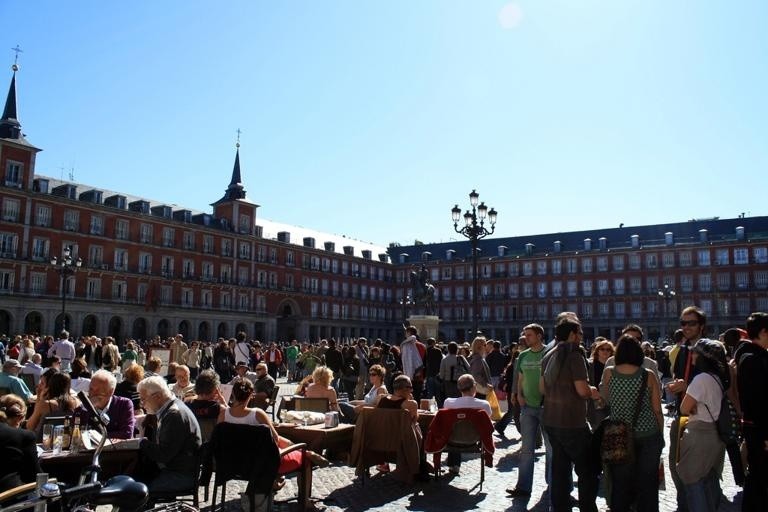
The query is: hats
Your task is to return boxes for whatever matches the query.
[233,361,250,371]
[687,338,723,361]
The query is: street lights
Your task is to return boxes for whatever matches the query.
[47,245,84,336]
[398,293,415,328]
[658,283,676,340]
[450,192,497,345]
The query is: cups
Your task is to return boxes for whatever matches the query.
[40,423,55,451]
[330,411,341,428]
[51,425,64,454]
[323,411,333,428]
[661,377,674,402]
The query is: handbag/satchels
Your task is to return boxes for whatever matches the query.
[600,421,636,466]
[498,375,507,393]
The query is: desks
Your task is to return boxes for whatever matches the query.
[416,409,445,478]
[277,395,294,422]
[273,423,356,464]
[38,440,143,480]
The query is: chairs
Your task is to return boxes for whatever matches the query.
[291,397,329,413]
[212,421,308,512]
[361,407,413,487]
[71,377,91,394]
[252,386,280,422]
[0,387,10,399]
[433,409,485,491]
[18,374,36,395]
[339,402,357,424]
[151,438,211,507]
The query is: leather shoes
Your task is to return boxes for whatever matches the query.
[506,489,531,497]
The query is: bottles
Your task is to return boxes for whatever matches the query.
[61,412,71,452]
[417,397,441,415]
[518,399,526,415]
[591,387,602,409]
[71,416,85,451]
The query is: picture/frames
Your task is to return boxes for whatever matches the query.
[148,347,172,377]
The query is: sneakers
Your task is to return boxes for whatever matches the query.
[376,464,390,472]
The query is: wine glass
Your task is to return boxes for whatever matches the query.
[302,408,309,429]
[279,407,288,425]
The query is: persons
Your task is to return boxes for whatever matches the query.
[1,305,768,512]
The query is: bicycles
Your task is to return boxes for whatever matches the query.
[0,389,200,512]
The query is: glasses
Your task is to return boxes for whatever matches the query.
[597,348,611,352]
[679,319,701,327]
[367,372,378,377]
[255,368,263,371]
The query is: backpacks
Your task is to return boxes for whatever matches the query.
[704,370,742,445]
